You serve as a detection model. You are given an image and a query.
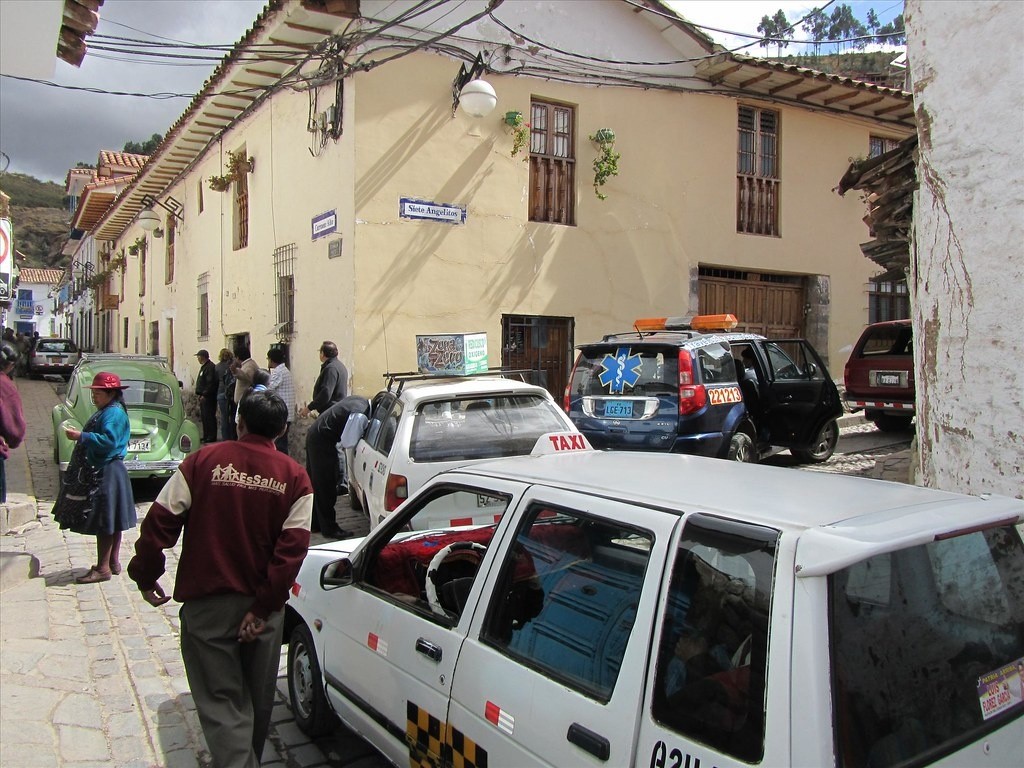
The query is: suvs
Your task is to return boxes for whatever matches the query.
[342,362,594,537]
[283,431,1024,768]
[843,319,916,432]
[27,337,83,378]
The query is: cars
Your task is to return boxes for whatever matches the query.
[50,350,200,491]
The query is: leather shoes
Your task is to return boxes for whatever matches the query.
[202,439,216,443]
[92,563,121,575]
[77,566,111,583]
[195,748,213,768]
[328,527,354,538]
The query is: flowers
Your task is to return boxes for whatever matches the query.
[510,115,532,163]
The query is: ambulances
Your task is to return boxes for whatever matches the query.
[560,314,845,463]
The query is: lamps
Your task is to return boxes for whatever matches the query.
[51,284,61,297]
[72,260,97,279]
[139,193,184,230]
[453,51,498,119]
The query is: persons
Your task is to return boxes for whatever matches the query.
[235,368,270,423]
[305,395,375,539]
[127,390,314,768]
[51,372,137,584]
[300,341,348,496]
[0,340,26,503]
[740,348,757,381]
[214,348,235,441]
[4,327,40,380]
[223,347,260,414]
[194,349,218,442]
[267,349,295,455]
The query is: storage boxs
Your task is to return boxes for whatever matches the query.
[415,331,488,377]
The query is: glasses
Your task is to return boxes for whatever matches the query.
[317,350,323,355]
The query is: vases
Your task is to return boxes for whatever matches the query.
[506,111,519,126]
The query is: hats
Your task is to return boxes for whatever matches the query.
[82,372,130,389]
[194,350,209,358]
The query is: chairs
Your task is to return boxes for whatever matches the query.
[461,403,505,428]
[419,412,433,433]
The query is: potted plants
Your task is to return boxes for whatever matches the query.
[589,127,622,201]
[128,245,139,255]
[208,150,255,192]
[135,237,147,250]
[153,228,164,238]
